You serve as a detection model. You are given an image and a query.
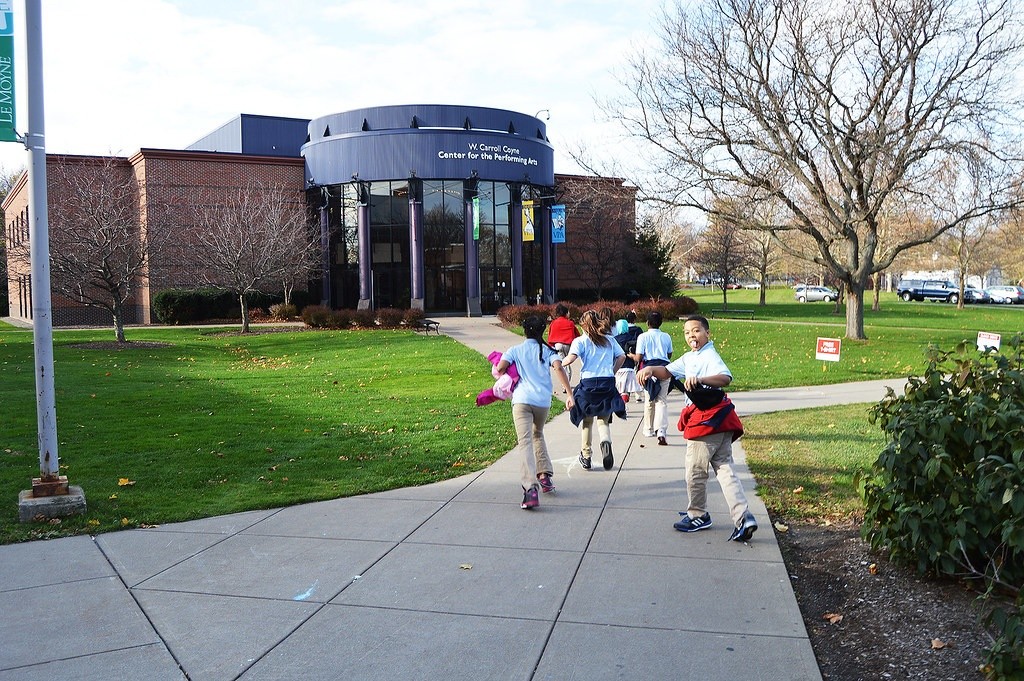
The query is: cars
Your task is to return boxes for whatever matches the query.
[695,275,767,289]
[958,283,1005,305]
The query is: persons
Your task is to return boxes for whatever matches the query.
[627,312,673,446]
[553,309,626,470]
[548,304,581,393]
[636,315,758,542]
[612,313,644,403]
[497,316,575,508]
[597,308,616,340]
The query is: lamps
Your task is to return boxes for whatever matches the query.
[470,169,480,179]
[408,169,417,178]
[306,178,317,187]
[523,171,532,183]
[351,173,364,182]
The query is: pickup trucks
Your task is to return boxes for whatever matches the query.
[896,279,974,305]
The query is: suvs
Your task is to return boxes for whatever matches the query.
[984,285,1024,305]
[794,285,844,303]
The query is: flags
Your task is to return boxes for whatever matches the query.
[522,200,534,241]
[473,197,480,240]
[552,204,565,242]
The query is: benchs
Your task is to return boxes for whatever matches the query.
[417,319,440,337]
[711,308,755,320]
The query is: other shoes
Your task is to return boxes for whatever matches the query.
[636,398,644,404]
[561,386,567,394]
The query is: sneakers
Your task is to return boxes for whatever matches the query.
[673,512,713,532]
[642,429,655,438]
[600,440,614,471]
[538,474,556,494]
[519,485,540,509]
[578,451,592,470]
[727,512,758,543]
[656,428,668,445]
